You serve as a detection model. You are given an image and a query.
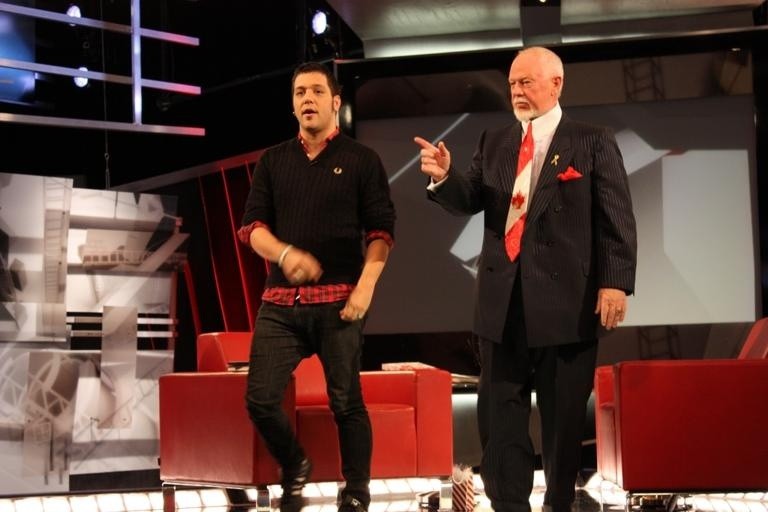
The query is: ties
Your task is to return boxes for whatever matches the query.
[504,123,533,263]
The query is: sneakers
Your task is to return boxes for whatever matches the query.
[280,457,309,512]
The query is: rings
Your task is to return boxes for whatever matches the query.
[615,309,622,314]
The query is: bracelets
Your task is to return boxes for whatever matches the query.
[276,242,294,272]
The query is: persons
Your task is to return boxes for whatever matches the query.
[413,46,635,512]
[239,63,395,512]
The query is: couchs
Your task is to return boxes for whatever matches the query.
[592,314,768,512]
[154,328,455,512]
